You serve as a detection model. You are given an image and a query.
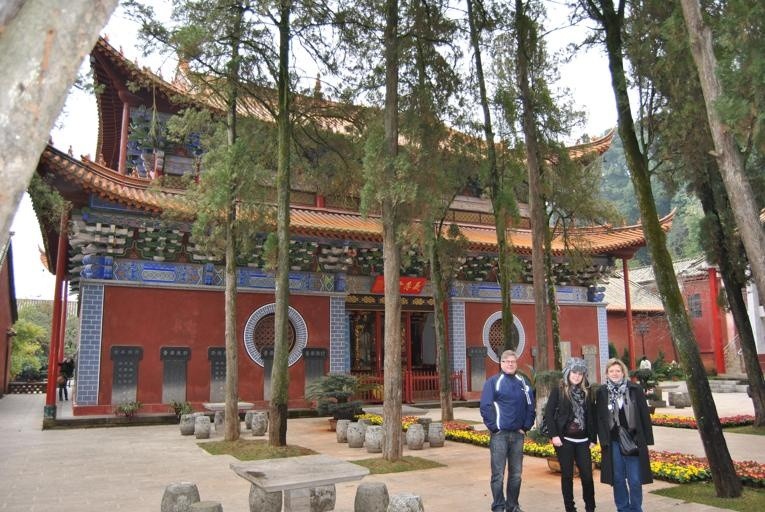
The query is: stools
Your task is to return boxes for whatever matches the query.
[213,409,226,430]
[669,390,692,408]
[243,410,267,437]
[161,481,225,512]
[247,478,338,512]
[334,419,443,454]
[178,412,213,438]
[352,481,425,512]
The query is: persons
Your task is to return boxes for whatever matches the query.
[478,349,537,512]
[592,357,656,512]
[544,356,597,512]
[639,354,653,372]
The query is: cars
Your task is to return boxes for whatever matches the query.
[15,364,48,381]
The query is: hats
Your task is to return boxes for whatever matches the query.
[562,358,589,385]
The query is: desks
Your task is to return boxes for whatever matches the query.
[203,402,254,437]
[361,405,429,417]
[229,454,370,512]
[651,382,680,408]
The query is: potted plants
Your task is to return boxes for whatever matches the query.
[306,373,384,432]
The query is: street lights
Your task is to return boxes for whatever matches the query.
[635,321,650,358]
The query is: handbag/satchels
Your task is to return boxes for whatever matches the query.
[618,425,639,456]
